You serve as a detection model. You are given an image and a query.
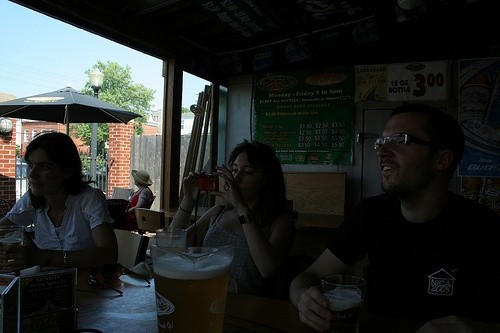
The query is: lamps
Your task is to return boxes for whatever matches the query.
[392,0,427,23]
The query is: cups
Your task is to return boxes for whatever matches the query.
[320,274,367,333]
[155,229,187,248]
[0,225,24,270]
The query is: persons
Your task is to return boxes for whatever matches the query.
[0,133,119,273]
[166,141,299,301]
[113,169,155,232]
[289,102,500,333]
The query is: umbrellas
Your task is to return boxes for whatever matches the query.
[0,82,142,136]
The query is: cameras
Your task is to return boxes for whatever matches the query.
[198,174,219,192]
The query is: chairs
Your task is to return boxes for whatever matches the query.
[113,228,149,270]
[134,207,166,235]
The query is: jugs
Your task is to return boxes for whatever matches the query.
[149,244,235,333]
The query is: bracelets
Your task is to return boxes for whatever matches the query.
[39,248,49,267]
[64,249,72,269]
[179,204,194,213]
[237,214,254,225]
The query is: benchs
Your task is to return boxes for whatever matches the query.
[281,172,347,229]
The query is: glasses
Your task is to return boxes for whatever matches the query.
[87,263,151,295]
[373,133,442,153]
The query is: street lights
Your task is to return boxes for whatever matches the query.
[86,72,105,188]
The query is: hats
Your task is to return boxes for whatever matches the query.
[131,170,153,186]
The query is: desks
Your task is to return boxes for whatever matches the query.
[77,265,418,333]
[164,207,214,220]
